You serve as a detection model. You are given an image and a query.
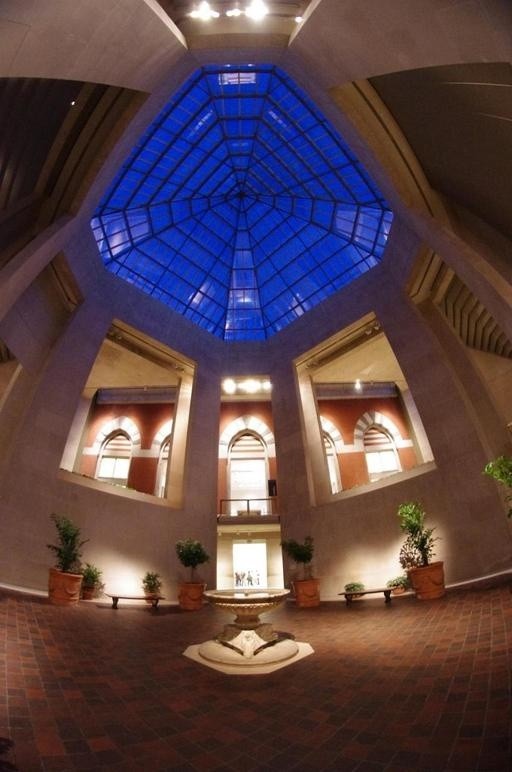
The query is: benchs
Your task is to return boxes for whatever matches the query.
[105,593,166,610]
[338,587,396,608]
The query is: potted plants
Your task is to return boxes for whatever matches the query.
[141,571,164,604]
[279,536,320,609]
[46,510,89,607]
[80,561,105,600]
[388,577,410,595]
[175,537,210,611]
[344,582,365,599]
[397,500,446,602]
